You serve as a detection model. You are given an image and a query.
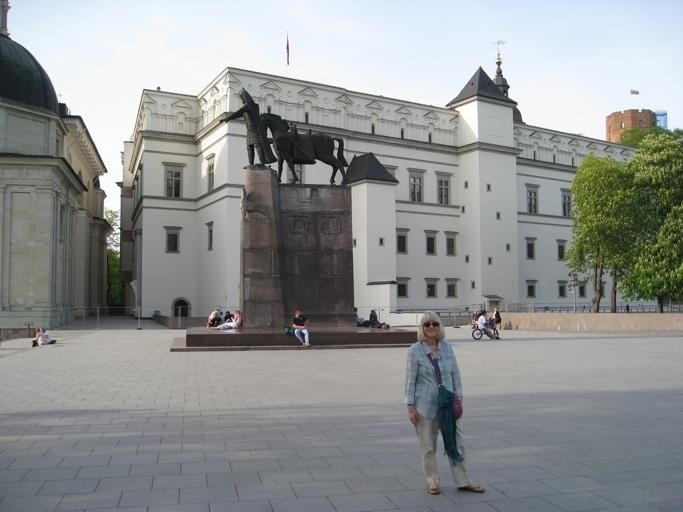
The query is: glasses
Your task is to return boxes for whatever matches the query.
[424,322,440,327]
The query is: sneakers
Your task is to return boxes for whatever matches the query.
[303,343,309,347]
[462,484,485,492]
[427,483,439,495]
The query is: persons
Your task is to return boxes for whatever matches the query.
[291,308,310,348]
[39,327,50,342]
[353,307,389,329]
[31,328,47,347]
[403,310,486,494]
[470,308,502,340]
[217,91,269,169]
[206,308,242,330]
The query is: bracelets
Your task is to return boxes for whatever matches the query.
[408,406,415,413]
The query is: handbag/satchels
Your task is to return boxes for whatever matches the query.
[438,386,463,418]
[284,326,294,335]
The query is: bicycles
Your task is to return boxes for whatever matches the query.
[471,317,499,340]
